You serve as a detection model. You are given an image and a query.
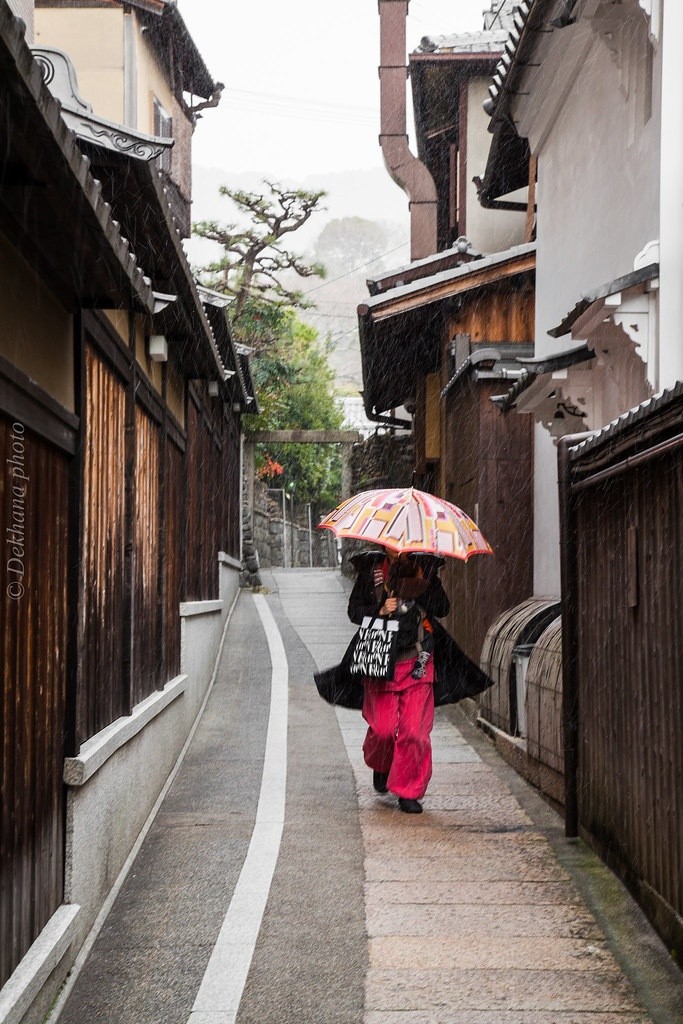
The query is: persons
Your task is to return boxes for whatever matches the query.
[384,559,435,680]
[314,544,495,813]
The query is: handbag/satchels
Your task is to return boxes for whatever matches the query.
[348,600,398,681]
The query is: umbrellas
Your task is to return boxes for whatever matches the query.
[319,485,494,596]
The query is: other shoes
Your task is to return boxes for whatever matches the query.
[398,797,422,814]
[373,769,389,793]
